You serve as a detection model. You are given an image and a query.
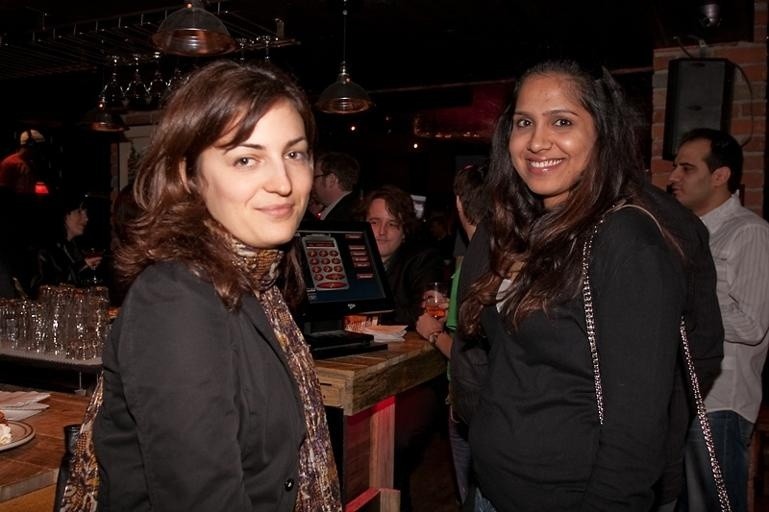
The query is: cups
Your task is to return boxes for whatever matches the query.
[0,281,112,360]
[422,281,450,317]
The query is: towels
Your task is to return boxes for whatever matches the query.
[0,390,51,422]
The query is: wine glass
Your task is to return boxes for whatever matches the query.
[81,248,104,284]
[92,32,283,131]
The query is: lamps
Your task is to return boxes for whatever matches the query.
[153,0,236,57]
[317,0,376,113]
[92,54,129,132]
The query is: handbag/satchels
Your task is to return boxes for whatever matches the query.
[53,425,100,512]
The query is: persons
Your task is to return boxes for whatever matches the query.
[62,55,347,512]
[447,47,732,510]
[304,150,497,512]
[669,127,769,512]
[1,126,138,306]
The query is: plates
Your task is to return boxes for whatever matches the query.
[0,419,35,452]
